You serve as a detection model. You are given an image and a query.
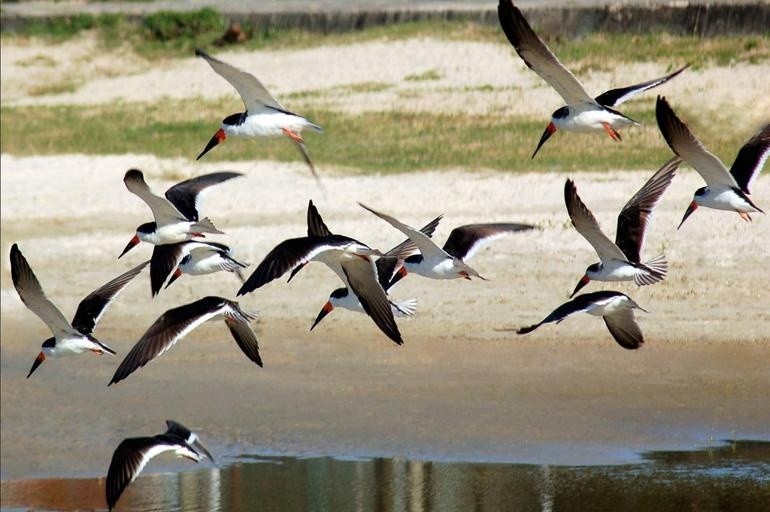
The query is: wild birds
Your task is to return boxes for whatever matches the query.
[358,201,542,280]
[308,212,445,345]
[107,296,263,386]
[195,49,323,181]
[498,0,693,159]
[238,197,384,295]
[117,169,250,298]
[10,243,152,378]
[516,291,650,349]
[656,94,770,230]
[565,156,682,298]
[106,420,214,512]
